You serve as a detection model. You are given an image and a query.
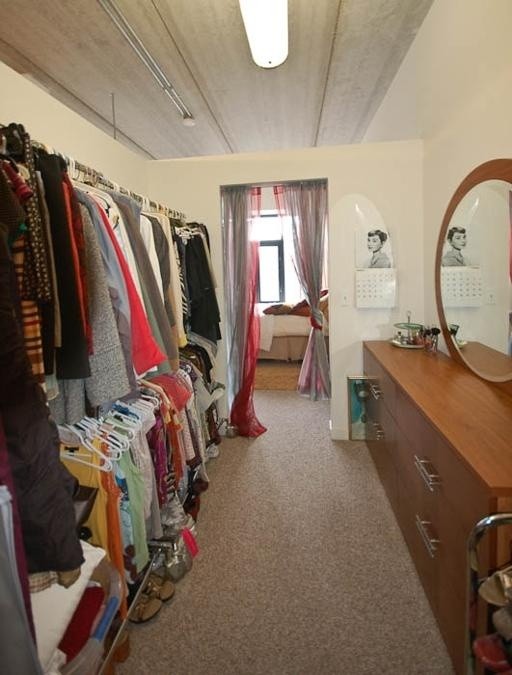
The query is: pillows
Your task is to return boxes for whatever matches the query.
[262,304,293,314]
[319,288,328,298]
[289,299,311,316]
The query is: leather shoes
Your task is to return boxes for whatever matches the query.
[472,565,511,674]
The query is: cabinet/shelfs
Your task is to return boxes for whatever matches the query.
[361,340,512,674]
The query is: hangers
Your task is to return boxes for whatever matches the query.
[1,142,190,222]
[60,384,162,473]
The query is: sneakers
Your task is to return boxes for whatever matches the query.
[129,573,174,623]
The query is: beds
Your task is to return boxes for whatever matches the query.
[254,298,329,362]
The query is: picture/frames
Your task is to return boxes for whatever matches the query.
[434,157,511,397]
[347,375,366,443]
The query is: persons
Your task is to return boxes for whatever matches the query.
[443,225,471,265]
[367,230,392,268]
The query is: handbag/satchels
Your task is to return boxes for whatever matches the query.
[160,495,188,526]
